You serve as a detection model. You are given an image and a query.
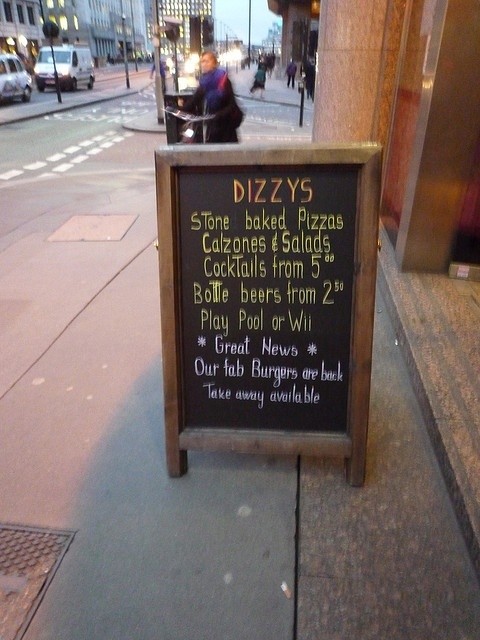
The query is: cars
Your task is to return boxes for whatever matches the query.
[1,54,32,103]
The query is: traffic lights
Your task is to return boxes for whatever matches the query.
[189,14,216,52]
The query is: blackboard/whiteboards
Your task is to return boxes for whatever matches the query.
[152,141,382,454]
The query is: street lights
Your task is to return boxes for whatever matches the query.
[43,20,60,38]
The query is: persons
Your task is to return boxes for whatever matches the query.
[240,53,314,102]
[150,55,166,96]
[168,51,243,142]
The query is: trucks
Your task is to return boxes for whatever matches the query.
[33,46,95,91]
